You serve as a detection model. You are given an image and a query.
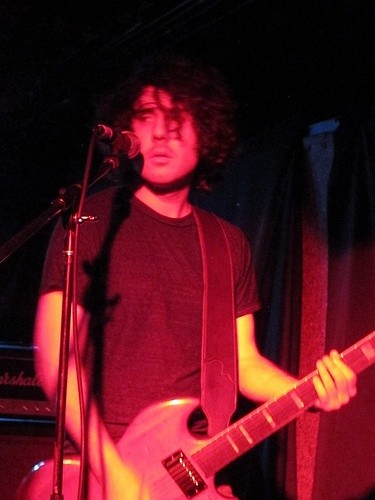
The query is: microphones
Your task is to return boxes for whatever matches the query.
[94,124,141,159]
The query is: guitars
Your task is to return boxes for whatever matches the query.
[15,329,375,500]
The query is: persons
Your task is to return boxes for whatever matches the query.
[34,65,356,500]
